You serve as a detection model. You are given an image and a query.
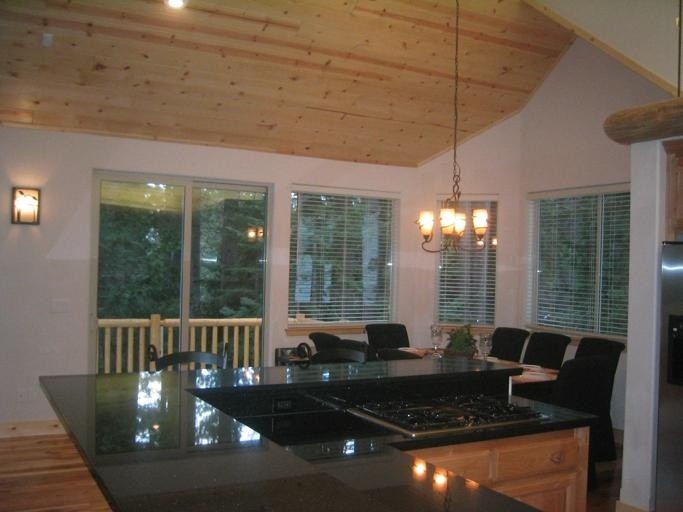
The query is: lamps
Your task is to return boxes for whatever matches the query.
[245,225,264,244]
[11,187,40,225]
[416,0,489,254]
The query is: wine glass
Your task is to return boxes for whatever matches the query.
[430,324,443,361]
[479,332,494,366]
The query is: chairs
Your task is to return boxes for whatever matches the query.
[365,323,409,349]
[319,339,372,363]
[514,331,571,399]
[529,354,607,476]
[309,332,340,352]
[574,337,625,461]
[378,347,421,359]
[148,341,232,371]
[488,327,529,363]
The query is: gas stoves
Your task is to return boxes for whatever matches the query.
[345,390,552,439]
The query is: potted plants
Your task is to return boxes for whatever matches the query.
[443,324,478,360]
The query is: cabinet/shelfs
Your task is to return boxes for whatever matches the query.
[403,426,590,512]
